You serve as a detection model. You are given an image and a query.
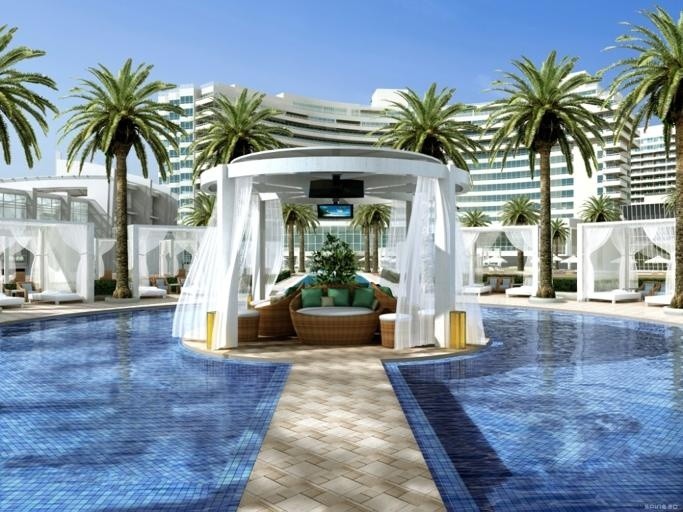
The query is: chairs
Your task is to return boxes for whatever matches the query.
[635,279,669,299]
[149,275,186,294]
[485,277,514,293]
[3,282,42,302]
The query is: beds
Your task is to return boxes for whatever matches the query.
[28,292,86,305]
[586,289,641,304]
[0,293,25,310]
[645,295,671,306]
[461,286,492,296]
[138,286,167,299]
[505,287,536,297]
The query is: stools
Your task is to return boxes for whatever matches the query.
[379,313,414,348]
[238,310,259,342]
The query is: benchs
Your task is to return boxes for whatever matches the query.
[254,279,397,346]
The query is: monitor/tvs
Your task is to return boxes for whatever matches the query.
[309,179,364,198]
[317,204,353,219]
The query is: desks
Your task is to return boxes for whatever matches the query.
[511,283,523,287]
